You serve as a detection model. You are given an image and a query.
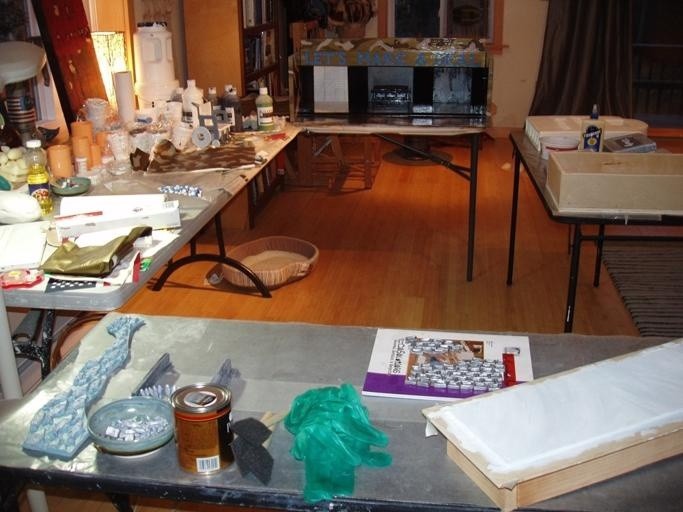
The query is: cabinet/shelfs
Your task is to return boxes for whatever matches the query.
[182,0,327,231]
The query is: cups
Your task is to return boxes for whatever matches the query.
[86,99,107,143]
[113,71,135,128]
[49,120,106,182]
[168,102,181,125]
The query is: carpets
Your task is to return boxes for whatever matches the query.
[594,238,683,339]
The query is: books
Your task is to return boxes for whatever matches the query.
[241,0,323,98]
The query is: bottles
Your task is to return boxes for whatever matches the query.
[255,87,273,131]
[26,139,53,213]
[175,80,240,132]
[579,104,604,153]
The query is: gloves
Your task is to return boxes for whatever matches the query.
[284,383,393,504]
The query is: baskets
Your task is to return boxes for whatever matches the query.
[222,237,319,291]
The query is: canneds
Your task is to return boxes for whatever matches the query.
[168,382,233,476]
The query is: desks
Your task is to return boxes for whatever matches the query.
[292,120,487,282]
[0,115,300,385]
[0,312,683,512]
[506,126,683,334]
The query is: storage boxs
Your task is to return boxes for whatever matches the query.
[546,150,683,211]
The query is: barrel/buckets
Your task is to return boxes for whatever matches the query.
[133,30,176,103]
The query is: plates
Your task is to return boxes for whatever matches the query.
[539,136,579,149]
[86,396,174,454]
[50,177,91,196]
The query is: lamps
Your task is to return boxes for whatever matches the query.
[92,31,130,111]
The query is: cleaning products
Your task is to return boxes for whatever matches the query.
[174,78,274,131]
